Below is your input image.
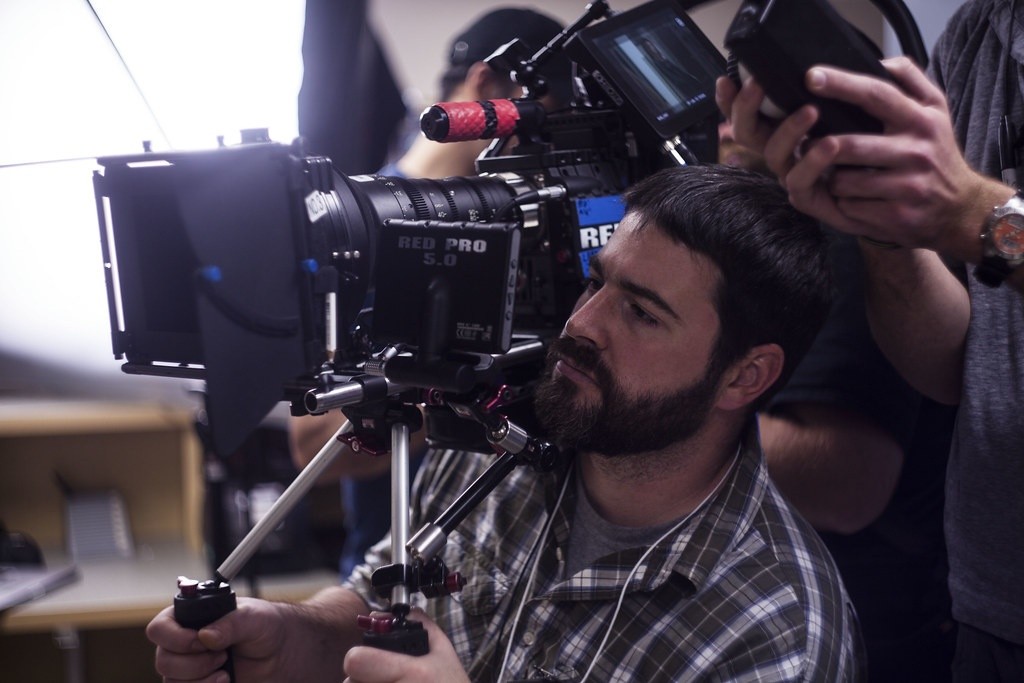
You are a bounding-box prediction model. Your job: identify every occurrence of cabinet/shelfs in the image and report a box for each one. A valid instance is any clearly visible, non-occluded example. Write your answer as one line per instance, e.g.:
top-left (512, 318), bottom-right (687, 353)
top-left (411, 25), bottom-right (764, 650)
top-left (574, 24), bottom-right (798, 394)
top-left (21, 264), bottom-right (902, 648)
top-left (0, 401), bottom-right (343, 683)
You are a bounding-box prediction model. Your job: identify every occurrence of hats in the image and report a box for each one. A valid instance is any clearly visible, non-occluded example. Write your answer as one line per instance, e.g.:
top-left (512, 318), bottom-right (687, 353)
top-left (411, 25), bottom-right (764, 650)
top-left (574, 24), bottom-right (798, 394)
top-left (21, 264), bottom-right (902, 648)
top-left (448, 8), bottom-right (567, 71)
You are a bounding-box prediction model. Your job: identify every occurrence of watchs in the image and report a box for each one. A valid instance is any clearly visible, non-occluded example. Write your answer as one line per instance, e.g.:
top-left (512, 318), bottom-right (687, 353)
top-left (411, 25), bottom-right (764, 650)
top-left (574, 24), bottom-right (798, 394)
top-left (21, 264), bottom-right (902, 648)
top-left (972, 188), bottom-right (1024, 289)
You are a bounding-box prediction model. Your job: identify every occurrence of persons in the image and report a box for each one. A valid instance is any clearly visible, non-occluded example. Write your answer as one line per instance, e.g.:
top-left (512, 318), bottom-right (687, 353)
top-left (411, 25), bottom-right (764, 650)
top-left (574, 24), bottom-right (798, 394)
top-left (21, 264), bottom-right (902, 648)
top-left (146, 164), bottom-right (861, 683)
top-left (715, 0), bottom-right (1024, 683)
top-left (287, 6), bottom-right (565, 585)
